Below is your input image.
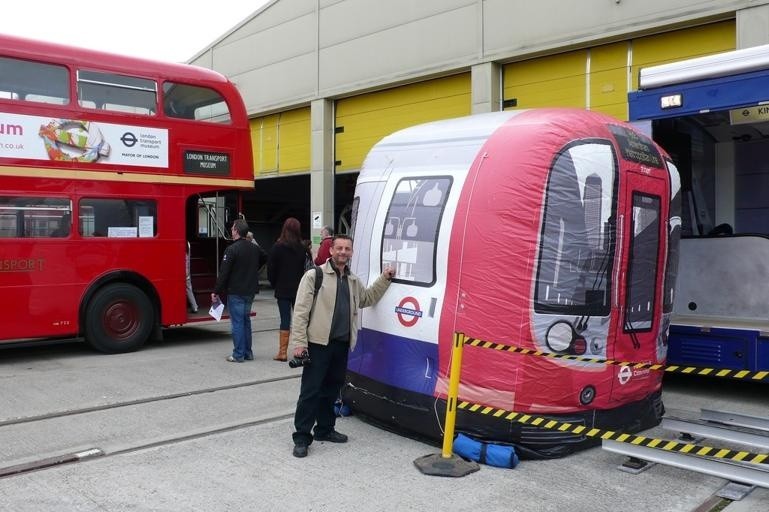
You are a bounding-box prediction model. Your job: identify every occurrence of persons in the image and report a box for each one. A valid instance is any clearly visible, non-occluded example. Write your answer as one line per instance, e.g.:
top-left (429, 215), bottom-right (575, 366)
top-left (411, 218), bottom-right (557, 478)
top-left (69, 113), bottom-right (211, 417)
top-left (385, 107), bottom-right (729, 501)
top-left (209, 218), bottom-right (265, 362)
top-left (245, 231), bottom-right (259, 249)
top-left (186, 240), bottom-right (199, 316)
top-left (49, 213), bottom-right (71, 238)
top-left (291, 236), bottom-right (395, 460)
top-left (314, 224), bottom-right (335, 264)
top-left (266, 213), bottom-right (310, 360)
top-left (304, 238), bottom-right (314, 269)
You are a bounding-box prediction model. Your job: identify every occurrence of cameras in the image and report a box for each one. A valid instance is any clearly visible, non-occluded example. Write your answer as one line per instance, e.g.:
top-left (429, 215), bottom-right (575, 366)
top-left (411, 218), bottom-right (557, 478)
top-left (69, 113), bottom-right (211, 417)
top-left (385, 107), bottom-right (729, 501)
top-left (289, 352), bottom-right (311, 368)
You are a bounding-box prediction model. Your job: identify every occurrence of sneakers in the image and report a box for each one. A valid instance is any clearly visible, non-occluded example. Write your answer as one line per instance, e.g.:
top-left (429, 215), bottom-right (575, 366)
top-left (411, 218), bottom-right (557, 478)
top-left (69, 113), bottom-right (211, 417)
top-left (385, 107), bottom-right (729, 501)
top-left (226, 355), bottom-right (238, 362)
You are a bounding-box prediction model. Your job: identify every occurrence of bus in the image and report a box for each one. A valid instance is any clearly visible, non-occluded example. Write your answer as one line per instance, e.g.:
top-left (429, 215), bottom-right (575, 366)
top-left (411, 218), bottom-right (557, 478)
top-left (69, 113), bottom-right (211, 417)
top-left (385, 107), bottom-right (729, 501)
top-left (0, 30), bottom-right (260, 357)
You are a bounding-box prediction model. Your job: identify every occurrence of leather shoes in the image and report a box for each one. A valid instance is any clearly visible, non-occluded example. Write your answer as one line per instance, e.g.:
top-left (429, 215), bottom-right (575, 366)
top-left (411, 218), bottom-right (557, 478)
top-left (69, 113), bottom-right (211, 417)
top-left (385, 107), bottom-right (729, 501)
top-left (313, 428), bottom-right (348, 443)
top-left (293, 443), bottom-right (308, 457)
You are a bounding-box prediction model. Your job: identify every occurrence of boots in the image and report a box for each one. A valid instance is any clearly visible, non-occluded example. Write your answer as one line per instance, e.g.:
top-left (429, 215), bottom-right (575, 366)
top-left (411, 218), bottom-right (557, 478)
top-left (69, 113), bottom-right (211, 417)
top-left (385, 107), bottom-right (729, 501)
top-left (273, 329), bottom-right (290, 362)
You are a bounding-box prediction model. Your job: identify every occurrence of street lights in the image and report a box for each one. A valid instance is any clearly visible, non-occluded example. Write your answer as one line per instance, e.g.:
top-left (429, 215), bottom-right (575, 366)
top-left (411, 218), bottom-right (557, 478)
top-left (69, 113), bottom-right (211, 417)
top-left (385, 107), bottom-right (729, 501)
top-left (383, 215), bottom-right (421, 277)
